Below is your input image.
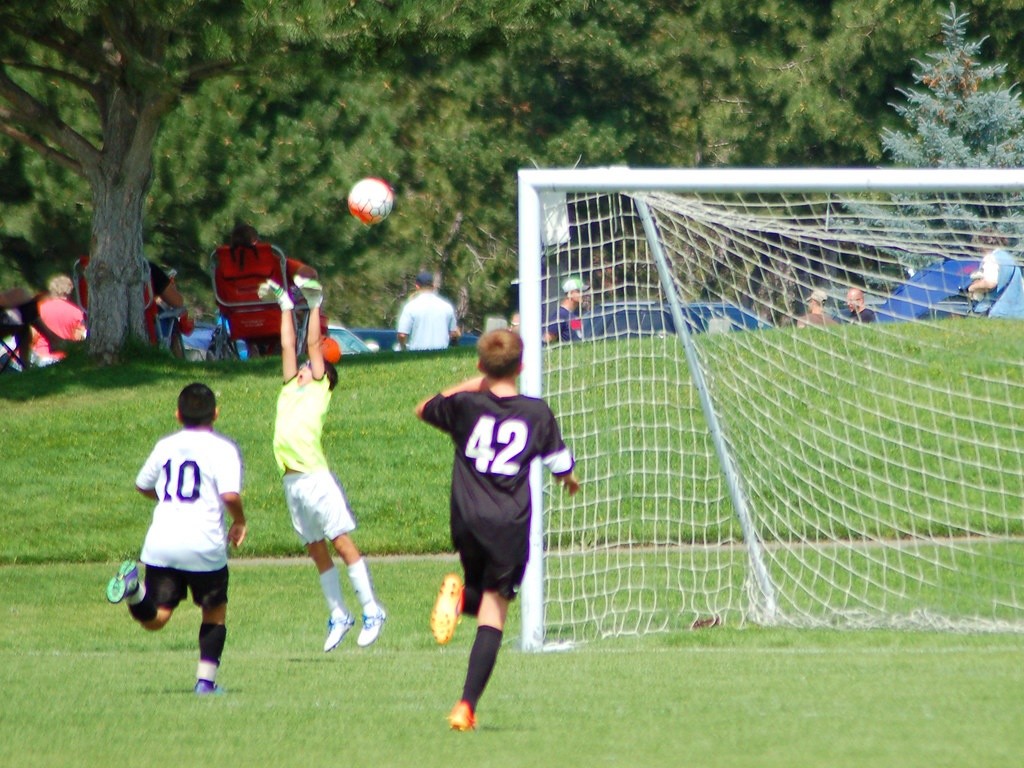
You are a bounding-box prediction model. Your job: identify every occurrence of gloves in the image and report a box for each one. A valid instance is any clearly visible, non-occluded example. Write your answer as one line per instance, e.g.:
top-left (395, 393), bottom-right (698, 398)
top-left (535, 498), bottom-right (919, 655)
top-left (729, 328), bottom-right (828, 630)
top-left (258, 279), bottom-right (294, 312)
top-left (293, 274), bottom-right (324, 307)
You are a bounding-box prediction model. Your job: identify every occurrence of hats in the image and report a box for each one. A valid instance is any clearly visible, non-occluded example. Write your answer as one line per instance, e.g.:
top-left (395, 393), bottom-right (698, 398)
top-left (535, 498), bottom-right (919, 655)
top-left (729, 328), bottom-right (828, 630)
top-left (806, 291), bottom-right (827, 303)
top-left (415, 271), bottom-right (433, 286)
top-left (562, 278), bottom-right (591, 293)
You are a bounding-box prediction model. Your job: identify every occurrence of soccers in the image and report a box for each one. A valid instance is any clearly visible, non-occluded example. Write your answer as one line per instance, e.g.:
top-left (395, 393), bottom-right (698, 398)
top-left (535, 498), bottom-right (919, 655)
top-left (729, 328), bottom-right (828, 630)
top-left (347, 177), bottom-right (394, 225)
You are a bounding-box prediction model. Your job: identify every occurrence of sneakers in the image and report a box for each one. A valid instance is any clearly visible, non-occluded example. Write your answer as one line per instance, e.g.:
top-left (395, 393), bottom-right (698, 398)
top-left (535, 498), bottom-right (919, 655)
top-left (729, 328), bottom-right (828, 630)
top-left (194, 679), bottom-right (228, 699)
top-left (323, 610), bottom-right (355, 653)
top-left (357, 606), bottom-right (386, 647)
top-left (429, 573), bottom-right (465, 644)
top-left (444, 701), bottom-right (478, 735)
top-left (105, 559), bottom-right (139, 604)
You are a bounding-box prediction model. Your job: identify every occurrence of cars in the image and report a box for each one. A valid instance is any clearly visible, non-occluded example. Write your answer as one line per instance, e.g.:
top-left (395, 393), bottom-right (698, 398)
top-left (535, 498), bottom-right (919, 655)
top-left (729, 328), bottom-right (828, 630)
top-left (557, 254), bottom-right (979, 341)
top-left (183, 322), bottom-right (482, 361)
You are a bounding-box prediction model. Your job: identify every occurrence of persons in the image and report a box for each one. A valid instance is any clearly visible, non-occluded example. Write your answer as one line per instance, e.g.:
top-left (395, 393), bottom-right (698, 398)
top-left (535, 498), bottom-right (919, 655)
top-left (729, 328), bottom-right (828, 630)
top-left (148, 262), bottom-right (184, 340)
top-left (107, 383), bottom-right (248, 694)
top-left (397, 273), bottom-right (462, 349)
top-left (257, 274), bottom-right (387, 653)
top-left (967, 224), bottom-right (1024, 319)
top-left (846, 288), bottom-right (874, 323)
top-left (14, 276), bottom-right (86, 372)
top-left (414, 329), bottom-right (579, 732)
top-left (543, 278), bottom-right (591, 343)
top-left (797, 291), bottom-right (837, 328)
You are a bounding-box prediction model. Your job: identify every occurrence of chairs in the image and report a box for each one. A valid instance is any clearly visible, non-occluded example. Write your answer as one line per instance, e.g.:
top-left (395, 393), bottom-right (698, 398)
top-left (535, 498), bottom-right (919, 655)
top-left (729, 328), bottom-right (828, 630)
top-left (209, 243), bottom-right (329, 359)
top-left (1, 257), bottom-right (187, 374)
